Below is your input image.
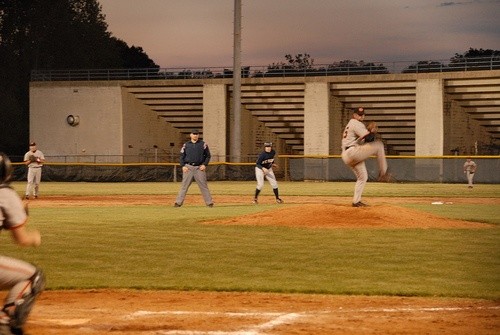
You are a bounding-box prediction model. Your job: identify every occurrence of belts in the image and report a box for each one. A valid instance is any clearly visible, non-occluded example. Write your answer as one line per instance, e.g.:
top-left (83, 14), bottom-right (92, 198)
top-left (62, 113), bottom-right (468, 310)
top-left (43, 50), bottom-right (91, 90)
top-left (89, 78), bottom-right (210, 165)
top-left (33, 167), bottom-right (40, 168)
top-left (184, 162), bottom-right (199, 166)
top-left (346, 147), bottom-right (349, 149)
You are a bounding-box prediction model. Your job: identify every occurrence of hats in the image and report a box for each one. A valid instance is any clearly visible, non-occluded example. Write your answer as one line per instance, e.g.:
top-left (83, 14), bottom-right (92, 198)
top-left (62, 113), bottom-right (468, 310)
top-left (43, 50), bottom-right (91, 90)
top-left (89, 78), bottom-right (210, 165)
top-left (29, 142), bottom-right (35, 145)
top-left (354, 107), bottom-right (366, 114)
top-left (192, 129), bottom-right (199, 133)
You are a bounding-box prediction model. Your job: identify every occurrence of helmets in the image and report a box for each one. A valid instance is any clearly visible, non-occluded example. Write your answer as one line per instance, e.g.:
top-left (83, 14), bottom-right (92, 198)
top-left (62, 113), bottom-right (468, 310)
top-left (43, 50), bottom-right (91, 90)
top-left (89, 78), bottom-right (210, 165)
top-left (264, 141), bottom-right (273, 147)
top-left (0, 152), bottom-right (13, 184)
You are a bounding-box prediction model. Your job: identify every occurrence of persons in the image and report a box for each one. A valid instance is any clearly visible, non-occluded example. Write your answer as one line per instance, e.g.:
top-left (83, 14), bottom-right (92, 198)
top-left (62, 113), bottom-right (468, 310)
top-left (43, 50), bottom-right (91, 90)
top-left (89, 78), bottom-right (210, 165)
top-left (463, 157), bottom-right (476, 188)
top-left (252, 142), bottom-right (282, 205)
top-left (0, 152), bottom-right (46, 335)
top-left (174, 130), bottom-right (214, 208)
top-left (341, 107), bottom-right (394, 207)
top-left (24, 142), bottom-right (45, 200)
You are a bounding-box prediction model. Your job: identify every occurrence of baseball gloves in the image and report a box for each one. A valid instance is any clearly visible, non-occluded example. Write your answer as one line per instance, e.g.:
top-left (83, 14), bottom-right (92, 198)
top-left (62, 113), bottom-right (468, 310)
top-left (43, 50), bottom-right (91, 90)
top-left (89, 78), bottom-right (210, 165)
top-left (29, 154), bottom-right (36, 161)
top-left (272, 163), bottom-right (279, 172)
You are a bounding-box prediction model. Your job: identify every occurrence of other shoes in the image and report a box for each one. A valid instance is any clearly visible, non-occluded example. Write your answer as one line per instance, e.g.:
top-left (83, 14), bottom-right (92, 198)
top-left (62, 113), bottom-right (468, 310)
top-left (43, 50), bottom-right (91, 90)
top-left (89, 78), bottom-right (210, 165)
top-left (0, 326), bottom-right (23, 335)
top-left (253, 200), bottom-right (258, 203)
top-left (376, 175), bottom-right (392, 180)
top-left (276, 198), bottom-right (282, 203)
top-left (34, 195), bottom-right (37, 198)
top-left (208, 203), bottom-right (213, 207)
top-left (174, 203), bottom-right (181, 207)
top-left (26, 195), bottom-right (29, 199)
top-left (352, 201), bottom-right (366, 207)
top-left (468, 186), bottom-right (473, 188)
